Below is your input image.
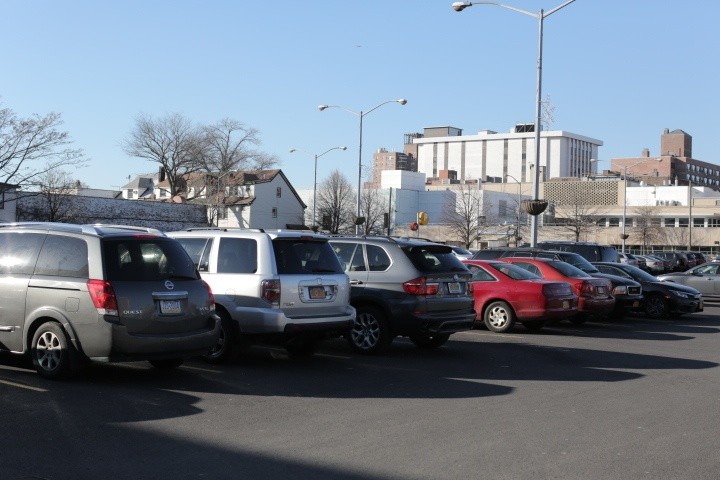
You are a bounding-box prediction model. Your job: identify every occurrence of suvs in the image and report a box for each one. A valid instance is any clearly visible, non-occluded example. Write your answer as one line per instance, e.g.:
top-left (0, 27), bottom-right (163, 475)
top-left (304, 233), bottom-right (478, 357)
top-left (470, 245), bottom-right (646, 325)
top-left (120, 226), bottom-right (357, 357)
top-left (0, 221), bottom-right (221, 383)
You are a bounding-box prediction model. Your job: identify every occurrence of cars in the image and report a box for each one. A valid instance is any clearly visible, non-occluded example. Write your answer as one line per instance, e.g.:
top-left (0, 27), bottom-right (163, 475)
top-left (572, 261), bottom-right (705, 321)
top-left (462, 259), bottom-right (580, 333)
top-left (423, 240), bottom-right (720, 277)
top-left (654, 259), bottom-right (720, 302)
top-left (473, 256), bottom-right (616, 326)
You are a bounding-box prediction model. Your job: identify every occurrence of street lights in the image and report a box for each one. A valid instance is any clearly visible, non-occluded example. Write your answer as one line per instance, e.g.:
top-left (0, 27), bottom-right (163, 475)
top-left (216, 170), bottom-right (238, 228)
top-left (504, 173), bottom-right (521, 247)
top-left (288, 145), bottom-right (347, 226)
top-left (452, 0), bottom-right (578, 247)
top-left (591, 157), bottom-right (663, 253)
top-left (318, 98), bottom-right (408, 234)
